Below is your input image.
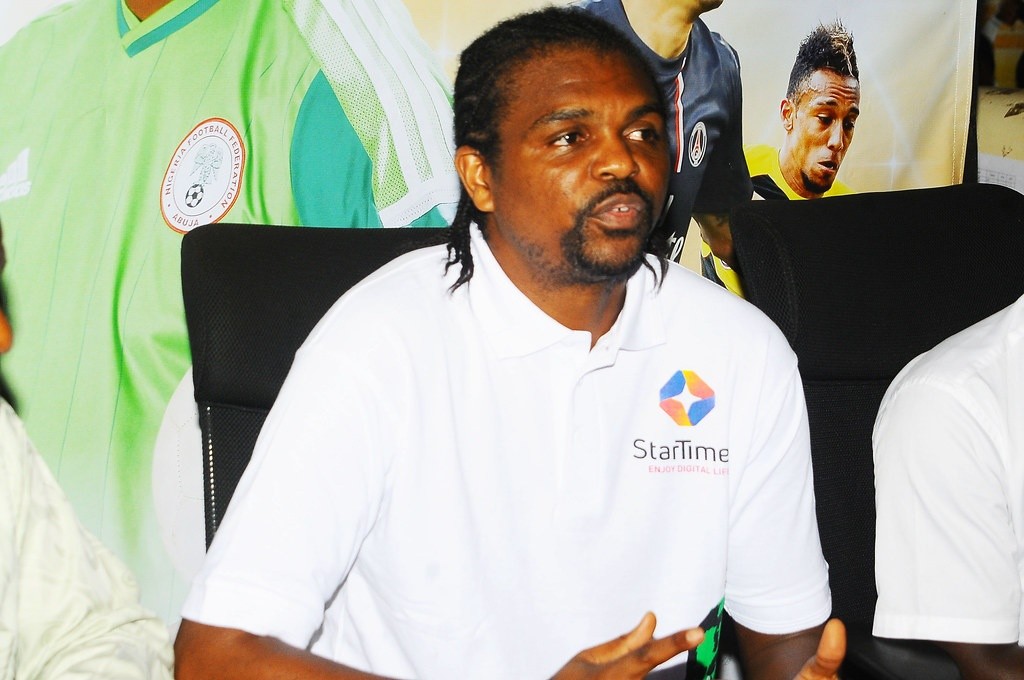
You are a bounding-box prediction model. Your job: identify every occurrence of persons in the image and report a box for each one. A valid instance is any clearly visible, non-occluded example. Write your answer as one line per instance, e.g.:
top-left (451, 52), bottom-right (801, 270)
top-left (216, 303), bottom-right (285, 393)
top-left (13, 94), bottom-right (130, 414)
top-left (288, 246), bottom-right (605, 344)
top-left (0, 306), bottom-right (174, 680)
top-left (872, 294), bottom-right (1024, 680)
top-left (0, 0), bottom-right (861, 631)
top-left (173, 7), bottom-right (846, 680)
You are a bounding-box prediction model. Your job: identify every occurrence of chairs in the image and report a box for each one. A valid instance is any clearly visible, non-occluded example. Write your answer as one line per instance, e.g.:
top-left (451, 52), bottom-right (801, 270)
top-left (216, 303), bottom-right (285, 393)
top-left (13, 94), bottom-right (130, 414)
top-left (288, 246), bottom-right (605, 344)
top-left (723, 183), bottom-right (1024, 680)
top-left (180, 222), bottom-right (465, 556)
